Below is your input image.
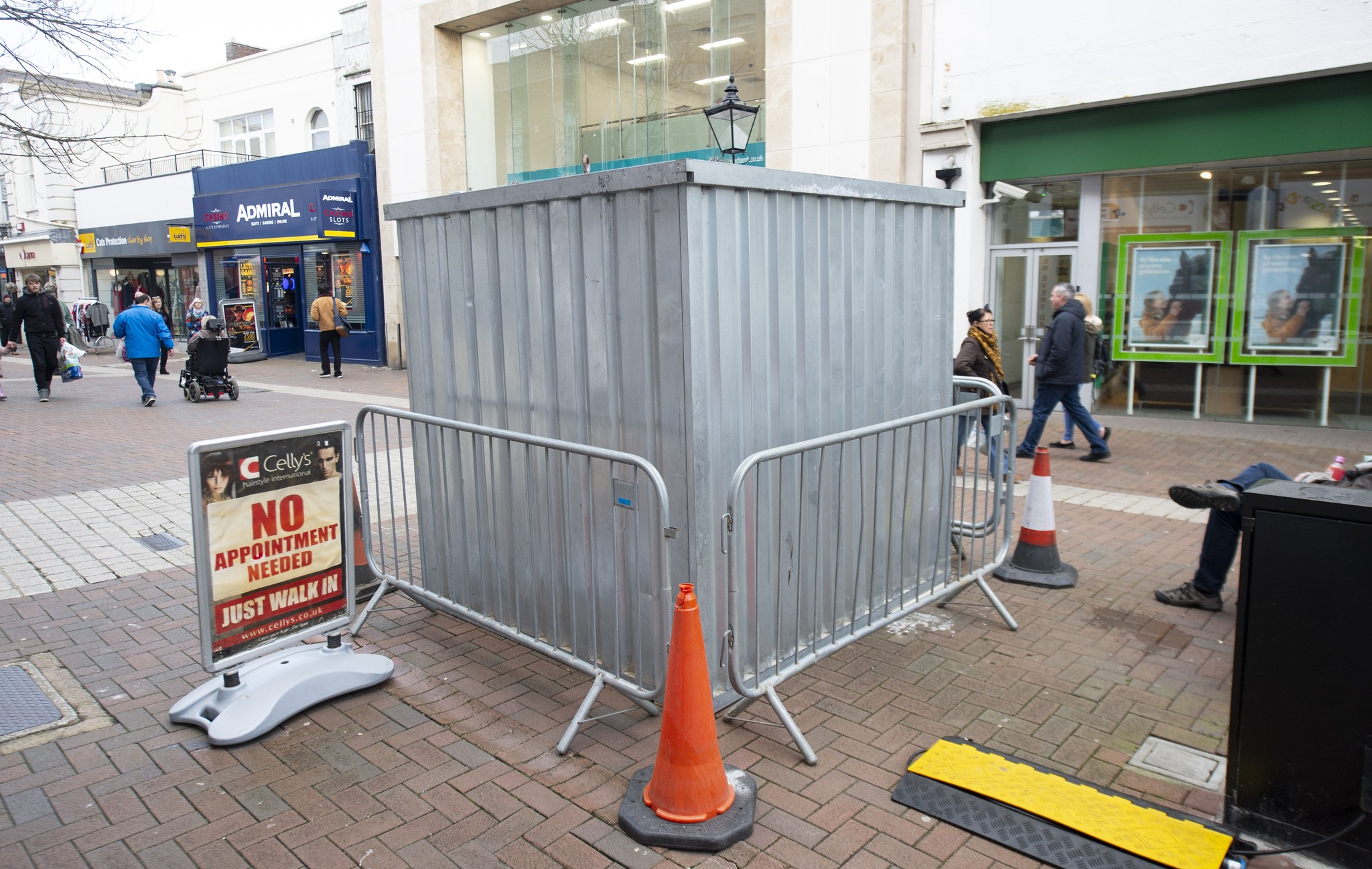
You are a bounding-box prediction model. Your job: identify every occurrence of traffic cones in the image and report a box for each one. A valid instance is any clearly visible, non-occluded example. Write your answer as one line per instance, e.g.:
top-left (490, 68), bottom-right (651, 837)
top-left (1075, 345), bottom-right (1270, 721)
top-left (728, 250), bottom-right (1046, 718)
top-left (618, 584), bottom-right (757, 852)
top-left (993, 446), bottom-right (1078, 589)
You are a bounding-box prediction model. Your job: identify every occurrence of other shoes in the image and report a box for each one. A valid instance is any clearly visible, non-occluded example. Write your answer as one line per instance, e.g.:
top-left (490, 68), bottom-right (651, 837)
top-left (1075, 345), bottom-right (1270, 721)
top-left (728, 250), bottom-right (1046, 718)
top-left (160, 367), bottom-right (170, 375)
top-left (1004, 447), bottom-right (1034, 459)
top-left (953, 468), bottom-right (963, 476)
top-left (1090, 427), bottom-right (1111, 449)
top-left (992, 475), bottom-right (1021, 484)
top-left (1080, 451), bottom-right (1111, 461)
top-left (1050, 441), bottom-right (1075, 450)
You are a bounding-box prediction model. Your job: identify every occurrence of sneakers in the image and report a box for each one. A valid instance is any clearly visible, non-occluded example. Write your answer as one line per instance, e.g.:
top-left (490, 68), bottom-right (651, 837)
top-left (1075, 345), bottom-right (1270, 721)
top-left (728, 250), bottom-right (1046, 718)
top-left (39, 389), bottom-right (49, 402)
top-left (318, 371), bottom-right (331, 377)
top-left (332, 373), bottom-right (342, 378)
top-left (143, 397), bottom-right (156, 406)
top-left (1155, 580), bottom-right (1223, 611)
top-left (1169, 483), bottom-right (1240, 512)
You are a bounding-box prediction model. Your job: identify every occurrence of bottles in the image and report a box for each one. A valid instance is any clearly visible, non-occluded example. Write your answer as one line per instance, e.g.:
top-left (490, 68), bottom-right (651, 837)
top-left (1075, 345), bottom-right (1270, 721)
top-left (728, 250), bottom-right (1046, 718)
top-left (1322, 455), bottom-right (1346, 482)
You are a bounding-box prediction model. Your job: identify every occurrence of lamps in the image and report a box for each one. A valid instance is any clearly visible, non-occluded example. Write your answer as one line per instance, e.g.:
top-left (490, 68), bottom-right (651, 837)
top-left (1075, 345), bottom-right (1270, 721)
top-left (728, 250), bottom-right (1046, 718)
top-left (110, 270), bottom-right (119, 276)
top-left (48, 269), bottom-right (59, 283)
top-left (16, 222), bottom-right (25, 232)
top-left (156, 270), bottom-right (164, 276)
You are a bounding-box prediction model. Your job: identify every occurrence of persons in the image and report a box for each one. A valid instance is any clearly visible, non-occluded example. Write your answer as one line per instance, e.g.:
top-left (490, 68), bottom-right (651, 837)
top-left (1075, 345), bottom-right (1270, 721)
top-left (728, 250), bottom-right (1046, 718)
top-left (311, 283), bottom-right (348, 378)
top-left (1262, 290), bottom-right (1310, 355)
top-left (1005, 282), bottom-right (1112, 462)
top-left (126, 292), bottom-right (174, 374)
top-left (311, 438), bottom-right (343, 483)
top-left (44, 288), bottom-right (75, 375)
top-left (200, 452), bottom-right (236, 515)
top-left (952, 308), bottom-right (1022, 483)
top-left (113, 294), bottom-right (174, 407)
top-left (1140, 290), bottom-right (1182, 351)
top-left (0, 294), bottom-right (19, 355)
top-left (187, 315), bottom-right (231, 380)
top-left (231, 305), bottom-right (246, 323)
top-left (121, 281), bottom-right (133, 309)
top-left (186, 298), bottom-right (210, 347)
top-left (0, 343), bottom-right (13, 400)
top-left (7, 273), bottom-right (67, 402)
top-left (1155, 454), bottom-right (1372, 611)
top-left (337, 256), bottom-right (351, 285)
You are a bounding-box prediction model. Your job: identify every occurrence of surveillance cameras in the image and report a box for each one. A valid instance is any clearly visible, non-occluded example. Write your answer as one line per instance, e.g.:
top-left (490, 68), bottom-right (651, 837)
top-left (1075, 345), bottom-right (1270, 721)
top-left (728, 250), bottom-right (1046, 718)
top-left (991, 179), bottom-right (1031, 200)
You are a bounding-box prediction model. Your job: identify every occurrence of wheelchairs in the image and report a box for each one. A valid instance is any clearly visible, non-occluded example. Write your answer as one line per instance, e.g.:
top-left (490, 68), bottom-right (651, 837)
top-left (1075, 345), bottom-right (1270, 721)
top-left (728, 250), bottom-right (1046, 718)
top-left (179, 318), bottom-right (239, 403)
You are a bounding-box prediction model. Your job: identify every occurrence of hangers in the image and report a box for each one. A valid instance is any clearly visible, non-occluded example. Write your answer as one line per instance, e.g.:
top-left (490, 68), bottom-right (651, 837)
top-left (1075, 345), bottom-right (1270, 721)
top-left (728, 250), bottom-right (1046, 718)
top-left (75, 296), bottom-right (101, 307)
top-left (161, 278), bottom-right (174, 287)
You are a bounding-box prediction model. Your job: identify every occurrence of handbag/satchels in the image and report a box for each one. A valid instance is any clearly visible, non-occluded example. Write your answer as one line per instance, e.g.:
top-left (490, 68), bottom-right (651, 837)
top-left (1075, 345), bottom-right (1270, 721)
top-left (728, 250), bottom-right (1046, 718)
top-left (334, 315), bottom-right (353, 338)
top-left (115, 337), bottom-right (129, 364)
top-left (58, 341), bottom-right (86, 383)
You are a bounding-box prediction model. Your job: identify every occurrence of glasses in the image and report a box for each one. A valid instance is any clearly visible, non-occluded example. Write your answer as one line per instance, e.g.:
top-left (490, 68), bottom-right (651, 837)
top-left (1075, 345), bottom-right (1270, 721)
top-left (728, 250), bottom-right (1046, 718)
top-left (978, 320), bottom-right (995, 324)
top-left (152, 296), bottom-right (160, 298)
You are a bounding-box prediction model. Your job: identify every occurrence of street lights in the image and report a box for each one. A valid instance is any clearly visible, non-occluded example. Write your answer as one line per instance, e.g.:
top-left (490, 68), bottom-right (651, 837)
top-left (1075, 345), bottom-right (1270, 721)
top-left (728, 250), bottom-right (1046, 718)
top-left (703, 71), bottom-right (760, 165)
